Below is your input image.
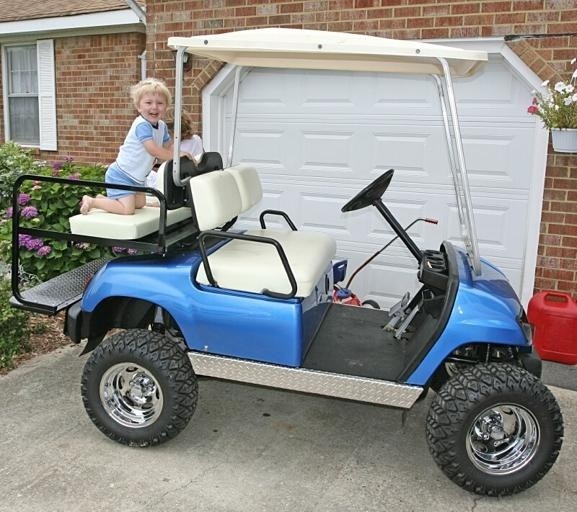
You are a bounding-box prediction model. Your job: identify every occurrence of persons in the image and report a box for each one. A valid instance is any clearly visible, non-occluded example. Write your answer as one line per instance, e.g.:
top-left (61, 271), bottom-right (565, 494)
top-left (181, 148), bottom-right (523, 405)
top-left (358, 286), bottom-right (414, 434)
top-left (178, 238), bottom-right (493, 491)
top-left (80, 78), bottom-right (197, 218)
top-left (146, 106), bottom-right (203, 207)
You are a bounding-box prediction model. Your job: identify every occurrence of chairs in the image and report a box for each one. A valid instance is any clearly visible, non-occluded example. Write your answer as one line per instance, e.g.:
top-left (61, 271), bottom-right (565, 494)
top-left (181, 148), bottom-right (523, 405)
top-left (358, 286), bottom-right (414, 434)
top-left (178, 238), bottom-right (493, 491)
top-left (69, 147), bottom-right (224, 239)
top-left (188, 162), bottom-right (337, 300)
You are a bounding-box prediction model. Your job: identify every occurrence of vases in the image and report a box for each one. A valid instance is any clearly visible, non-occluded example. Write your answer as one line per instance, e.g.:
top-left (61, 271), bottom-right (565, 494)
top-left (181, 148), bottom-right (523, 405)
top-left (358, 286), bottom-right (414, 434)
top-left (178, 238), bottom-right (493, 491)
top-left (551, 128), bottom-right (577, 153)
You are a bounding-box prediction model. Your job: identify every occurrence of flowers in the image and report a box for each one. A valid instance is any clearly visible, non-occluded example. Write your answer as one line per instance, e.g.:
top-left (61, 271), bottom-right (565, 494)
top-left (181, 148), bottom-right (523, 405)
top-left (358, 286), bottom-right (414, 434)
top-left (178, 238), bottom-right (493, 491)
top-left (526, 57), bottom-right (577, 130)
